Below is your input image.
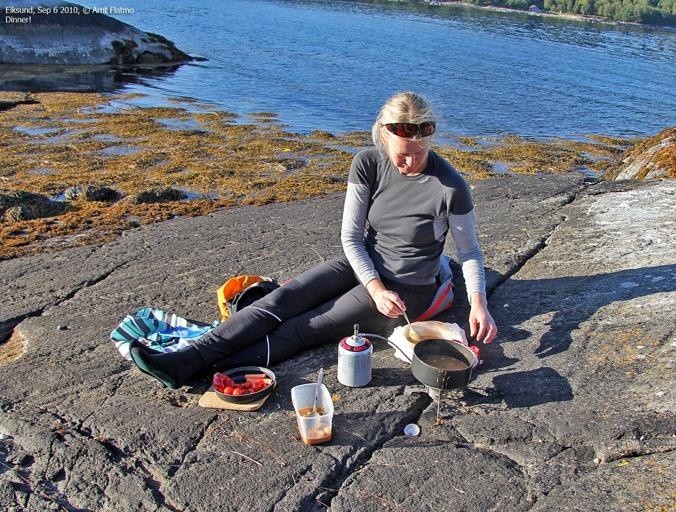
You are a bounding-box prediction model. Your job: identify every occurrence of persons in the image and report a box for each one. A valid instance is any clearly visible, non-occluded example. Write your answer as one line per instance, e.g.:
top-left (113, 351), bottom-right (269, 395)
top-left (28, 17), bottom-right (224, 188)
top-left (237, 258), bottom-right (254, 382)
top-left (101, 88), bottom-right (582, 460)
top-left (128, 90), bottom-right (500, 393)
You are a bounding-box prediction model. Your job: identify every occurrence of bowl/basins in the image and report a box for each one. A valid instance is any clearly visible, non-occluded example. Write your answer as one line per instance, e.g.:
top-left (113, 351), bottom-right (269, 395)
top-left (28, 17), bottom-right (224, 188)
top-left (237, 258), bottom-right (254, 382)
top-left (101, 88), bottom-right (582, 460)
top-left (410, 339), bottom-right (477, 389)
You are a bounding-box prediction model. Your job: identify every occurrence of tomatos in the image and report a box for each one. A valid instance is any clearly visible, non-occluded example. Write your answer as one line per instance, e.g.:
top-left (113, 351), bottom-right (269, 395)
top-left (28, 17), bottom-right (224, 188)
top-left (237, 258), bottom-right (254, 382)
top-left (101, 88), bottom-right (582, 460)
top-left (223, 380), bottom-right (266, 396)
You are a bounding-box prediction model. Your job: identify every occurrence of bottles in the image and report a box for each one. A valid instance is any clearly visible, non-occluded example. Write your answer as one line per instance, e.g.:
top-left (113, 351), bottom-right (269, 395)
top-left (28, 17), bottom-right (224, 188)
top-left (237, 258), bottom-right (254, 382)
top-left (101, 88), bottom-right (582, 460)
top-left (337, 324), bottom-right (372, 388)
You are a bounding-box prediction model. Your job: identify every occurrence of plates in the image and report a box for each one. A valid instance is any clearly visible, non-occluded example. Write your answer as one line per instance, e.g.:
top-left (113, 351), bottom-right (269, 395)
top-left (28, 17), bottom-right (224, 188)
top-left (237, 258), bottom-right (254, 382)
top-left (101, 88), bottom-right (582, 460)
top-left (212, 367), bottom-right (276, 403)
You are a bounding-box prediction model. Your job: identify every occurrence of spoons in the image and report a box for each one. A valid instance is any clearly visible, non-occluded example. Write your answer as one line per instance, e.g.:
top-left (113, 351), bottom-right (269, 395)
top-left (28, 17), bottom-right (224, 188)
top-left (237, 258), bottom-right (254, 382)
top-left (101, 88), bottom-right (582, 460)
top-left (305, 367), bottom-right (326, 424)
top-left (398, 300), bottom-right (423, 342)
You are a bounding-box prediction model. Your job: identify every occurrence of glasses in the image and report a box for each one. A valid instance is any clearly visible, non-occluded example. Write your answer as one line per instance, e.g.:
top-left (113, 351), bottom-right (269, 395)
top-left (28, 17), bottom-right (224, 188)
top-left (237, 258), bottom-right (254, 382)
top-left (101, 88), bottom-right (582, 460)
top-left (380, 120), bottom-right (437, 138)
